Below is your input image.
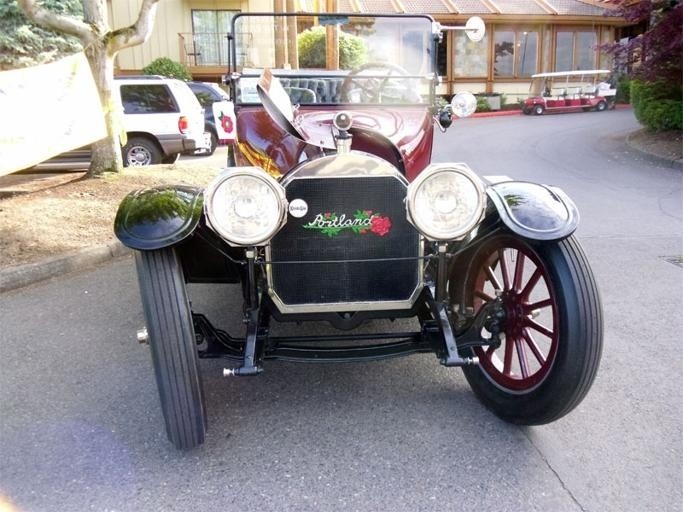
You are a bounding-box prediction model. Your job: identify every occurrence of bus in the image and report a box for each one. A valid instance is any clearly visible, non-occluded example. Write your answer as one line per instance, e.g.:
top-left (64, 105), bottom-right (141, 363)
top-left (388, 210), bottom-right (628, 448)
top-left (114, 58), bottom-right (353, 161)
top-left (521, 69), bottom-right (616, 114)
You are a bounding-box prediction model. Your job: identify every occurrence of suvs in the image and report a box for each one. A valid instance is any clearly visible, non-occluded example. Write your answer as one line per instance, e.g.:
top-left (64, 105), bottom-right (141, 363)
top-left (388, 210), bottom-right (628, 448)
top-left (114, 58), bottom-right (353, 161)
top-left (184, 80), bottom-right (232, 156)
top-left (114, 11), bottom-right (606, 455)
top-left (16, 76), bottom-right (211, 169)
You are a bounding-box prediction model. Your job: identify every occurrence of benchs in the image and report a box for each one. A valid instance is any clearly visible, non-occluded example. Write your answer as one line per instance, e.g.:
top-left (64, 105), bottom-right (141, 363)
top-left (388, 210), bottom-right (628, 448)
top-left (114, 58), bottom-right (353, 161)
top-left (548, 86), bottom-right (599, 100)
top-left (279, 78), bottom-right (421, 105)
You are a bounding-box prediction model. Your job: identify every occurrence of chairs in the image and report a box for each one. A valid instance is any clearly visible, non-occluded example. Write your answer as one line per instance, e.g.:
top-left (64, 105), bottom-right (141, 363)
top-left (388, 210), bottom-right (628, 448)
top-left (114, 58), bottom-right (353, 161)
top-left (283, 87), bottom-right (316, 104)
top-left (347, 87), bottom-right (420, 105)
